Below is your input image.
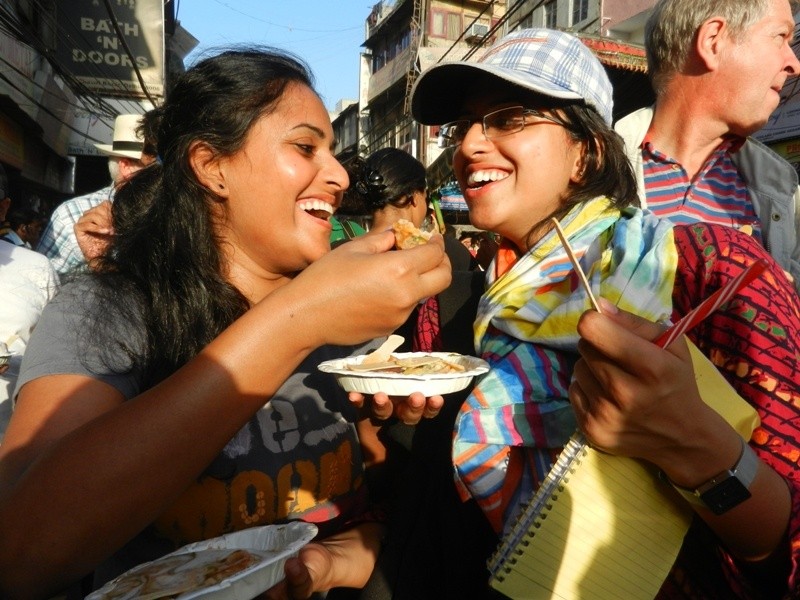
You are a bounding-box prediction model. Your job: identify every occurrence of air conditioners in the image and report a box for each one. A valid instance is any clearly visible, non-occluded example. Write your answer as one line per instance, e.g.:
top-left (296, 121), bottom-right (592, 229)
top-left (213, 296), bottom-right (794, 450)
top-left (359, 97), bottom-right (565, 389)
top-left (463, 23), bottom-right (489, 40)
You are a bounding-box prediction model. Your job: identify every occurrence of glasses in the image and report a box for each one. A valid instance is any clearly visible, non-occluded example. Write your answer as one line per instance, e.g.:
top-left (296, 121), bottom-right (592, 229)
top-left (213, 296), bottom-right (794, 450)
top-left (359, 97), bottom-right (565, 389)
top-left (435, 105), bottom-right (584, 149)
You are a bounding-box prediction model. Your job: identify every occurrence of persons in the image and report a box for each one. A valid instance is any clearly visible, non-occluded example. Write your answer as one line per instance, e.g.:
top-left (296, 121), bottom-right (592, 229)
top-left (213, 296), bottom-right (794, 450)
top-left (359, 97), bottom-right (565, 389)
top-left (0, 41), bottom-right (451, 600)
top-left (249, 26), bottom-right (800, 600)
top-left (0, 0), bottom-right (800, 600)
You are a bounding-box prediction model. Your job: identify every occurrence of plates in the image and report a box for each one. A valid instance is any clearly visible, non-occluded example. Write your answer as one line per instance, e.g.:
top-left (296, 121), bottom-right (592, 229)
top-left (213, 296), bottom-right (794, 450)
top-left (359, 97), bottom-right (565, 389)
top-left (86, 521), bottom-right (319, 599)
top-left (317, 352), bottom-right (492, 397)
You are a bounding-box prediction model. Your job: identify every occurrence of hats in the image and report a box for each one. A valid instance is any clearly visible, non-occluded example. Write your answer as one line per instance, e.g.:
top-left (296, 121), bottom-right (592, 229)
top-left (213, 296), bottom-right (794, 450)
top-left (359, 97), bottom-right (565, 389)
top-left (93, 114), bottom-right (148, 159)
top-left (408, 28), bottom-right (614, 130)
